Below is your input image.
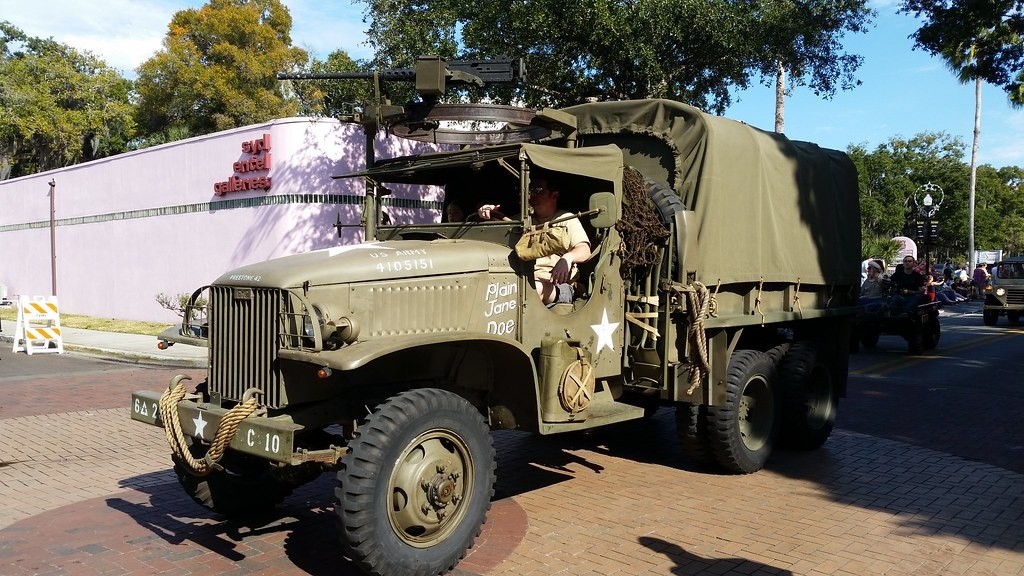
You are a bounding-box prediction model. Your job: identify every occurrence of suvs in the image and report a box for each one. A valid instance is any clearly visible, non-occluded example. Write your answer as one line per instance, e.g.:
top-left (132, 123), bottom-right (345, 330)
top-left (983, 256), bottom-right (1024, 325)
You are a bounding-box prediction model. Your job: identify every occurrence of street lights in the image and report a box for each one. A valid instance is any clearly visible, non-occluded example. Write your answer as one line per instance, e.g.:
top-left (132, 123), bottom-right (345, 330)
top-left (914, 181), bottom-right (945, 279)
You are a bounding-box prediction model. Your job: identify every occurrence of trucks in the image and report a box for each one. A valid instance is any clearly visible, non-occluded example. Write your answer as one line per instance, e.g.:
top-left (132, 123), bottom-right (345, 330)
top-left (131, 52), bottom-right (865, 576)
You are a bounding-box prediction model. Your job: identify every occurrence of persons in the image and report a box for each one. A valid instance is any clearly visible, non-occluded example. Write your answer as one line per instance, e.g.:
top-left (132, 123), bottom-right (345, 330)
top-left (861, 256), bottom-right (1024, 314)
top-left (445, 170), bottom-right (592, 305)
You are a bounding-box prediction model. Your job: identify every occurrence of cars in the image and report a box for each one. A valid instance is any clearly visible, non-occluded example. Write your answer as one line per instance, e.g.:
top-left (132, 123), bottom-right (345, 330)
top-left (933, 265), bottom-right (949, 277)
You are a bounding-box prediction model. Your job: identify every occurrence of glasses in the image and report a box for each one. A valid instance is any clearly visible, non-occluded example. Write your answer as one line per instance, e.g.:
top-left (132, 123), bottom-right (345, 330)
top-left (530, 186), bottom-right (549, 194)
top-left (867, 266), bottom-right (874, 270)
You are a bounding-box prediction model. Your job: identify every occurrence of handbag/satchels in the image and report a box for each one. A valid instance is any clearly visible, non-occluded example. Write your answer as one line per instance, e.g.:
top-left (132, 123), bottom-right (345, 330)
top-left (515, 221), bottom-right (570, 261)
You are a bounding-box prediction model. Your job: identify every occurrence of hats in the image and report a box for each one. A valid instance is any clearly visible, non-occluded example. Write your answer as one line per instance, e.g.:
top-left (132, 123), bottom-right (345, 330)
top-left (868, 260), bottom-right (884, 274)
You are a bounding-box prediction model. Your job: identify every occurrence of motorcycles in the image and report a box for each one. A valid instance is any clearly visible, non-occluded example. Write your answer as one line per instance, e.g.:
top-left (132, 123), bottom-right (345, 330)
top-left (865, 284), bottom-right (941, 354)
